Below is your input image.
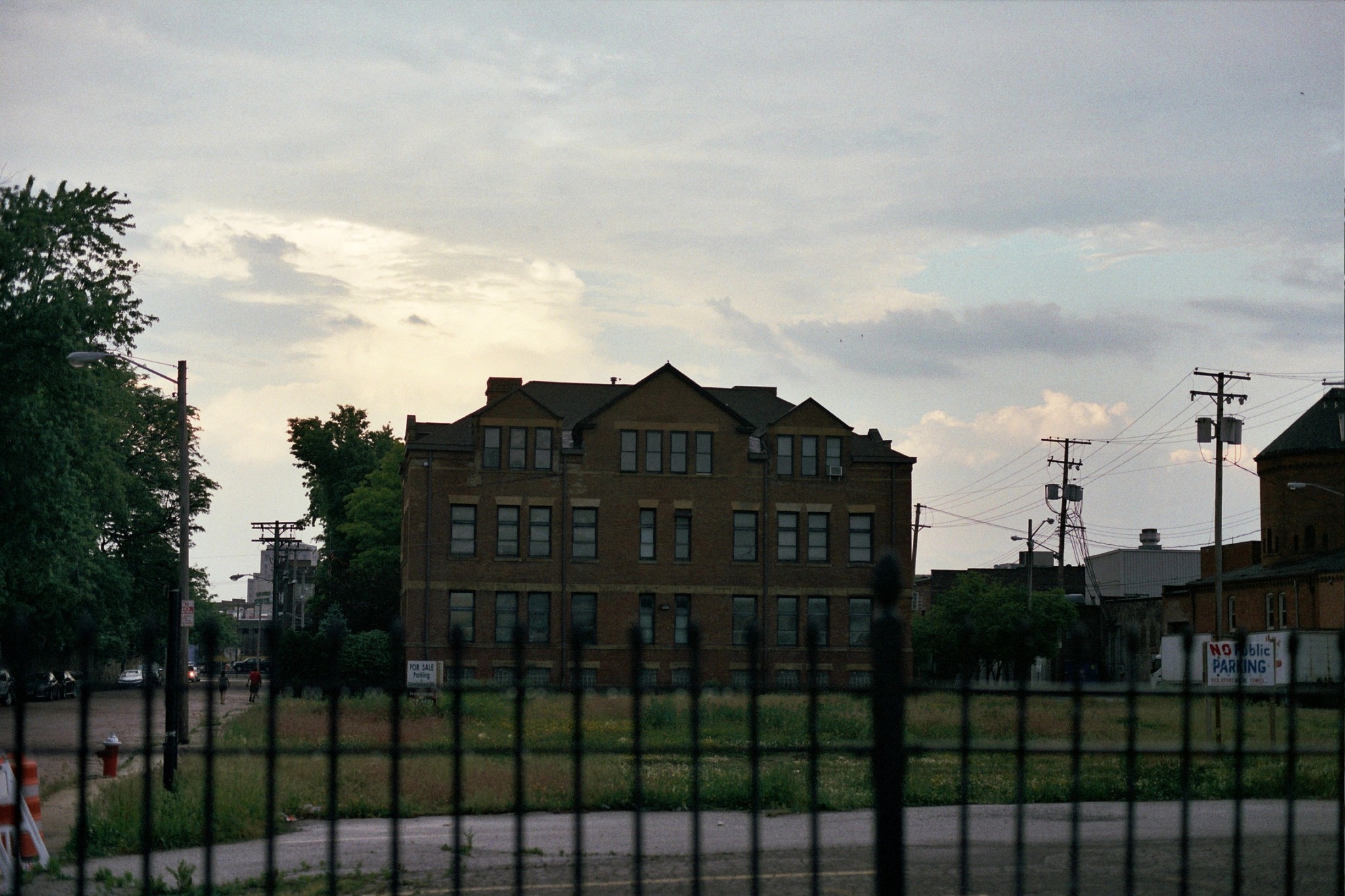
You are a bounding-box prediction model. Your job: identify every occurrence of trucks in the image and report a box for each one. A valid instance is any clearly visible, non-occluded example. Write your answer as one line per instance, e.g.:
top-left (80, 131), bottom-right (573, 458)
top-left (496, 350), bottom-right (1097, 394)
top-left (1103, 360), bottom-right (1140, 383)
top-left (1150, 633), bottom-right (1234, 686)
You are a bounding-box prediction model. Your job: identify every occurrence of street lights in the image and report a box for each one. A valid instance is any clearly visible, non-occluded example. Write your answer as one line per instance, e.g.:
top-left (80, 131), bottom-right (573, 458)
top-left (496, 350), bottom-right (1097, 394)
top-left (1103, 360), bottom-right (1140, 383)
top-left (1028, 517), bottom-right (1055, 613)
top-left (65, 350), bottom-right (187, 791)
top-left (279, 612), bottom-right (304, 629)
top-left (230, 573), bottom-right (278, 629)
top-left (1011, 535), bottom-right (1064, 595)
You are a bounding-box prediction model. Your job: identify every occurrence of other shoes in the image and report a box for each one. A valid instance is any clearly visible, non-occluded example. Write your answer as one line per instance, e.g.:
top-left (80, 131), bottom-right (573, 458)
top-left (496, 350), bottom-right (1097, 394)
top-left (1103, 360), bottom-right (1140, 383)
top-left (249, 697), bottom-right (252, 702)
top-left (252, 699), bottom-right (254, 702)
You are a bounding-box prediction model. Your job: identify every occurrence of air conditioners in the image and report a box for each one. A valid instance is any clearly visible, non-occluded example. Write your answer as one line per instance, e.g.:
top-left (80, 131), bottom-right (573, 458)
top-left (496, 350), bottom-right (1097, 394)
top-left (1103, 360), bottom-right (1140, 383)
top-left (826, 465), bottom-right (844, 477)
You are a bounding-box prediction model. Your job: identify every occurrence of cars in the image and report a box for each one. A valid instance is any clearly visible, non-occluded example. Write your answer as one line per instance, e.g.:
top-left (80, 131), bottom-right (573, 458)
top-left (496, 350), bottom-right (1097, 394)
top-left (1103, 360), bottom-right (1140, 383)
top-left (155, 668), bottom-right (162, 687)
top-left (117, 669), bottom-right (143, 687)
top-left (0, 670), bottom-right (77, 707)
top-left (188, 664), bottom-right (201, 682)
top-left (232, 657), bottom-right (270, 673)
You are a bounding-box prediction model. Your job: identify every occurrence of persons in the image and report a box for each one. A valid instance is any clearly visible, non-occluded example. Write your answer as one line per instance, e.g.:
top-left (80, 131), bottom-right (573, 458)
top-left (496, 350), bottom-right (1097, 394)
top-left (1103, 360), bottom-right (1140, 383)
top-left (246, 667), bottom-right (262, 703)
top-left (216, 670), bottom-right (230, 704)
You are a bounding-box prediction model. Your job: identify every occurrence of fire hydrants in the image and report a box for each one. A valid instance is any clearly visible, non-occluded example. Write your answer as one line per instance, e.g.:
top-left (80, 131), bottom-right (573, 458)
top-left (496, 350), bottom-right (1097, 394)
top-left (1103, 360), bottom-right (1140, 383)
top-left (95, 731), bottom-right (123, 778)
